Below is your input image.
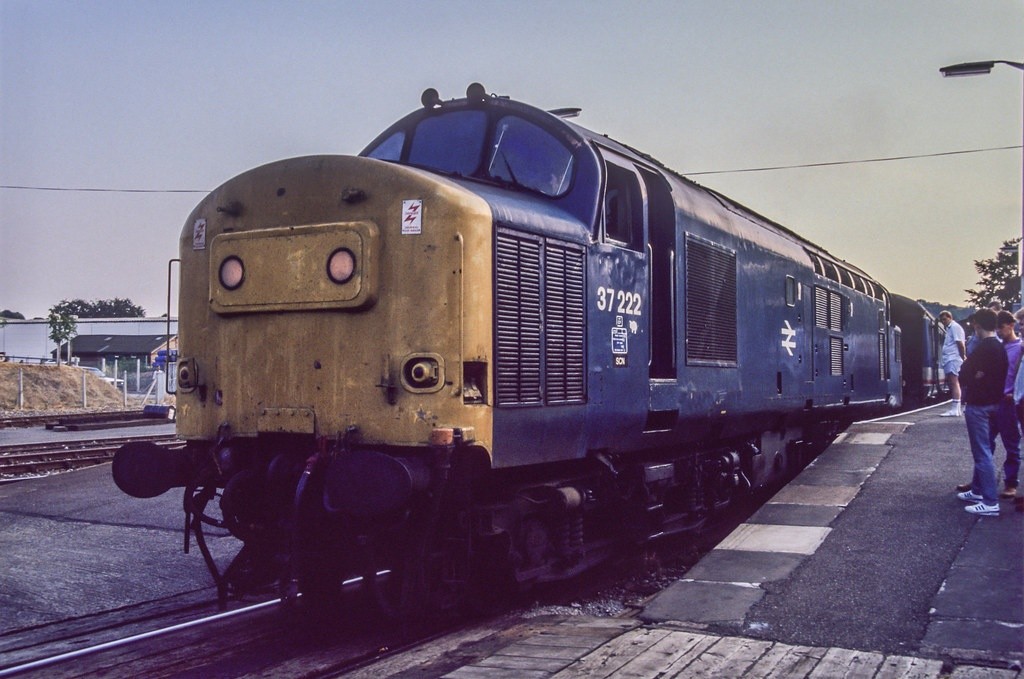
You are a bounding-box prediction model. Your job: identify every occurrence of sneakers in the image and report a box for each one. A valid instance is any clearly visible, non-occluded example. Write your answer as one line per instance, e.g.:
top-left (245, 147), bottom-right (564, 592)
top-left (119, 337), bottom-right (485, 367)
top-left (964, 501), bottom-right (1000, 516)
top-left (957, 490), bottom-right (983, 504)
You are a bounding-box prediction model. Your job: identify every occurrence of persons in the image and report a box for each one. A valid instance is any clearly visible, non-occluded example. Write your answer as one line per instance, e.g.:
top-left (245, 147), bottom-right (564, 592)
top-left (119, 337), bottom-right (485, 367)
top-left (957, 300), bottom-right (1024, 516)
top-left (152, 365), bottom-right (164, 380)
top-left (938, 310), bottom-right (967, 417)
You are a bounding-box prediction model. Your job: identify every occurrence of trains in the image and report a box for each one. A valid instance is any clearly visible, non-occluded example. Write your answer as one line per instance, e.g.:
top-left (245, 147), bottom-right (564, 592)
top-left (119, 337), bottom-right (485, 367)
top-left (110, 82), bottom-right (946, 616)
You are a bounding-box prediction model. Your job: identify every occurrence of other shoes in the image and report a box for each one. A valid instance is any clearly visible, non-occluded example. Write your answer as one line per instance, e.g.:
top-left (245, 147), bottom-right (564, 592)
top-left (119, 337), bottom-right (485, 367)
top-left (940, 408), bottom-right (961, 416)
top-left (957, 484), bottom-right (971, 492)
top-left (1001, 486), bottom-right (1016, 497)
